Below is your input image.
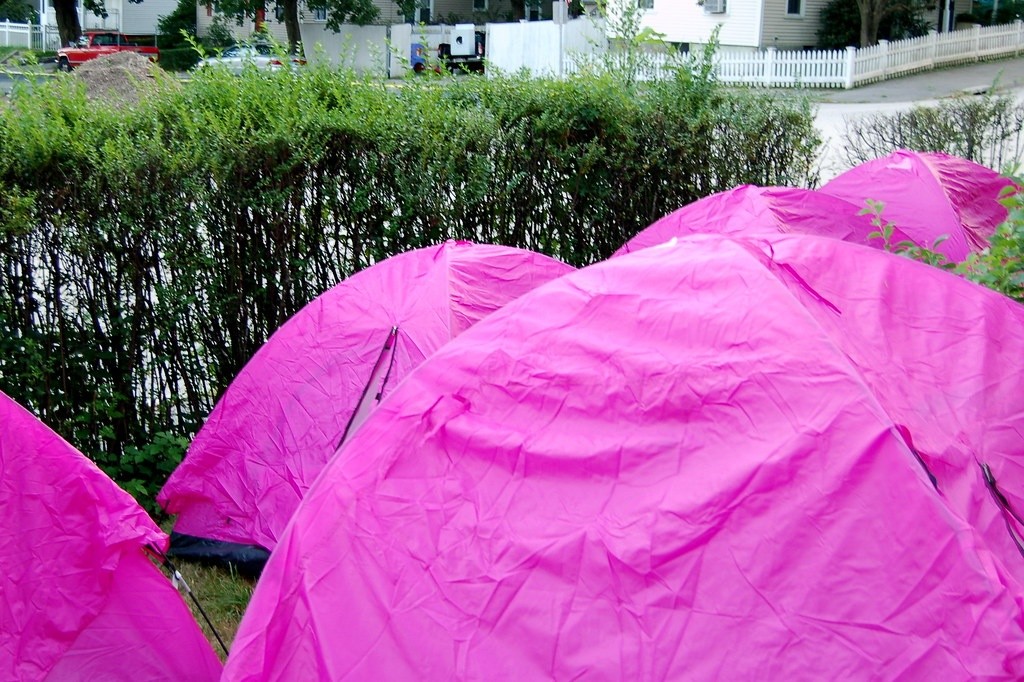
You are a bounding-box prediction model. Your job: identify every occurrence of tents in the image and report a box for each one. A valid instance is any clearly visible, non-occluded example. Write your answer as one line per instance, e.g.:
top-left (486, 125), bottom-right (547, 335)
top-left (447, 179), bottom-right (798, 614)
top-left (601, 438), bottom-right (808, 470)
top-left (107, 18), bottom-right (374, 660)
top-left (156, 148), bottom-right (1024, 682)
top-left (0, 388), bottom-right (230, 682)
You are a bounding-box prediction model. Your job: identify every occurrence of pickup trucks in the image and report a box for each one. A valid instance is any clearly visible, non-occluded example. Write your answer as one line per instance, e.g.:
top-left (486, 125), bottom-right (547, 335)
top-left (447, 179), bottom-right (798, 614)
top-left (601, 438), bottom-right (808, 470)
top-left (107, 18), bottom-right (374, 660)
top-left (438, 29), bottom-right (485, 75)
top-left (54, 30), bottom-right (159, 75)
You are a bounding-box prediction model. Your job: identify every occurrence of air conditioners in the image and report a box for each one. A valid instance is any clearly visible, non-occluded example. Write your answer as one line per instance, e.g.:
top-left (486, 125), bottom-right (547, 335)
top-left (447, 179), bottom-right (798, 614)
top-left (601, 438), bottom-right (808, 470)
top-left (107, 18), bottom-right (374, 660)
top-left (704, 0), bottom-right (724, 13)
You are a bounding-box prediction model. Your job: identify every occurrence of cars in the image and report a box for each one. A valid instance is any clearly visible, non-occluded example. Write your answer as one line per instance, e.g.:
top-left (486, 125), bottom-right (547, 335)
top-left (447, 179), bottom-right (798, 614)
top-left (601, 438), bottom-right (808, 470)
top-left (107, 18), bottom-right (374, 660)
top-left (195, 42), bottom-right (307, 81)
